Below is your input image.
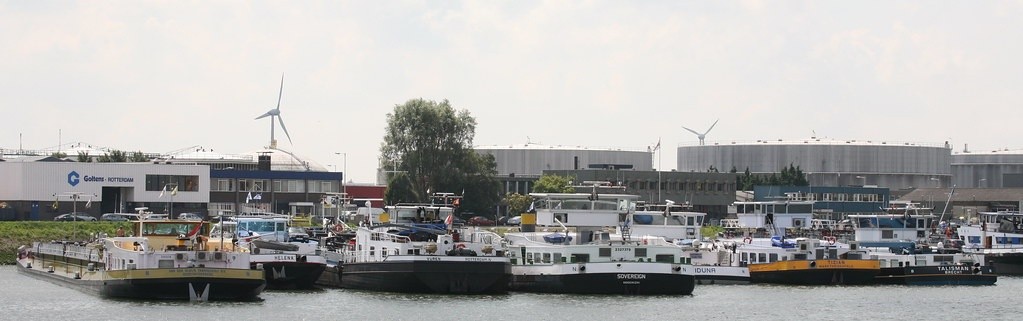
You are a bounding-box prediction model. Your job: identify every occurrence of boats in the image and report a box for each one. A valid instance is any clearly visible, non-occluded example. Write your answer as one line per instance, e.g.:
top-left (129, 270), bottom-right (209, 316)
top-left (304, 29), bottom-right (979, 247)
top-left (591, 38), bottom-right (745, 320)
top-left (130, 180), bottom-right (1023, 297)
top-left (13, 207), bottom-right (266, 303)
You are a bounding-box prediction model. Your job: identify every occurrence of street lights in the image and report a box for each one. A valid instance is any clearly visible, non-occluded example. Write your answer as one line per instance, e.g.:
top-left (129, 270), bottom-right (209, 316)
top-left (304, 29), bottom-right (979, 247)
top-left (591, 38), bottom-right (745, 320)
top-left (979, 178), bottom-right (987, 187)
top-left (931, 177), bottom-right (940, 187)
top-left (856, 175), bottom-right (866, 185)
top-left (335, 153), bottom-right (346, 203)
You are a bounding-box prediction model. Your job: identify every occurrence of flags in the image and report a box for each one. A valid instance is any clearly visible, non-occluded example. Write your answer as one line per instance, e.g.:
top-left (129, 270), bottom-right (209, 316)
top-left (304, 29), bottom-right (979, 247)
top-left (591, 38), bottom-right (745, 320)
top-left (85, 197), bottom-right (92, 209)
top-left (52, 197), bottom-right (58, 210)
top-left (171, 185), bottom-right (178, 196)
top-left (444, 215), bottom-right (453, 226)
top-left (246, 192), bottom-right (262, 204)
top-left (158, 185), bottom-right (166, 199)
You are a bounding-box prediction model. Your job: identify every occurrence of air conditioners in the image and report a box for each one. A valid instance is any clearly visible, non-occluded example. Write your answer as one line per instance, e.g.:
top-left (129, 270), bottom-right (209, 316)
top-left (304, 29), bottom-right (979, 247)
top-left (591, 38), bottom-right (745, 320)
top-left (212, 251), bottom-right (227, 261)
top-left (197, 251), bottom-right (210, 261)
top-left (174, 253), bottom-right (187, 261)
top-left (252, 248), bottom-right (266, 255)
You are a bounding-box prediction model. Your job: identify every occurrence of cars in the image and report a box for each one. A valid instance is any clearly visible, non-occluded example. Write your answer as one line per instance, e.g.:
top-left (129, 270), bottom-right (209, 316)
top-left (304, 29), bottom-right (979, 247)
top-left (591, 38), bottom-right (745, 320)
top-left (468, 216), bottom-right (496, 227)
top-left (506, 216), bottom-right (522, 226)
top-left (99, 212), bottom-right (130, 221)
top-left (178, 212), bottom-right (202, 222)
top-left (71, 212), bottom-right (97, 221)
top-left (54, 213), bottom-right (85, 222)
top-left (452, 216), bottom-right (467, 226)
top-left (496, 215), bottom-right (510, 227)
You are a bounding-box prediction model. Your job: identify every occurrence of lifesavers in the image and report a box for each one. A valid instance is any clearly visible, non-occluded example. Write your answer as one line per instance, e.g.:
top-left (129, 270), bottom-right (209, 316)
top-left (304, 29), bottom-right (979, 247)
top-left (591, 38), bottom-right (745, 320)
top-left (828, 236), bottom-right (836, 245)
top-left (457, 243), bottom-right (466, 249)
top-left (743, 237), bottom-right (752, 244)
top-left (335, 225), bottom-right (343, 232)
top-left (905, 213), bottom-right (911, 221)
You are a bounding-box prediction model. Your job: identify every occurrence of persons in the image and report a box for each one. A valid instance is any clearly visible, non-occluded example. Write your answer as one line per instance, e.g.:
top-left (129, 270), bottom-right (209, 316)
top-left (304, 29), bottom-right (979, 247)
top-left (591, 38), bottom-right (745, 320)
top-left (196, 234), bottom-right (208, 250)
top-left (284, 231), bottom-right (290, 242)
top-left (170, 228), bottom-right (178, 236)
top-left (116, 227), bottom-right (124, 237)
